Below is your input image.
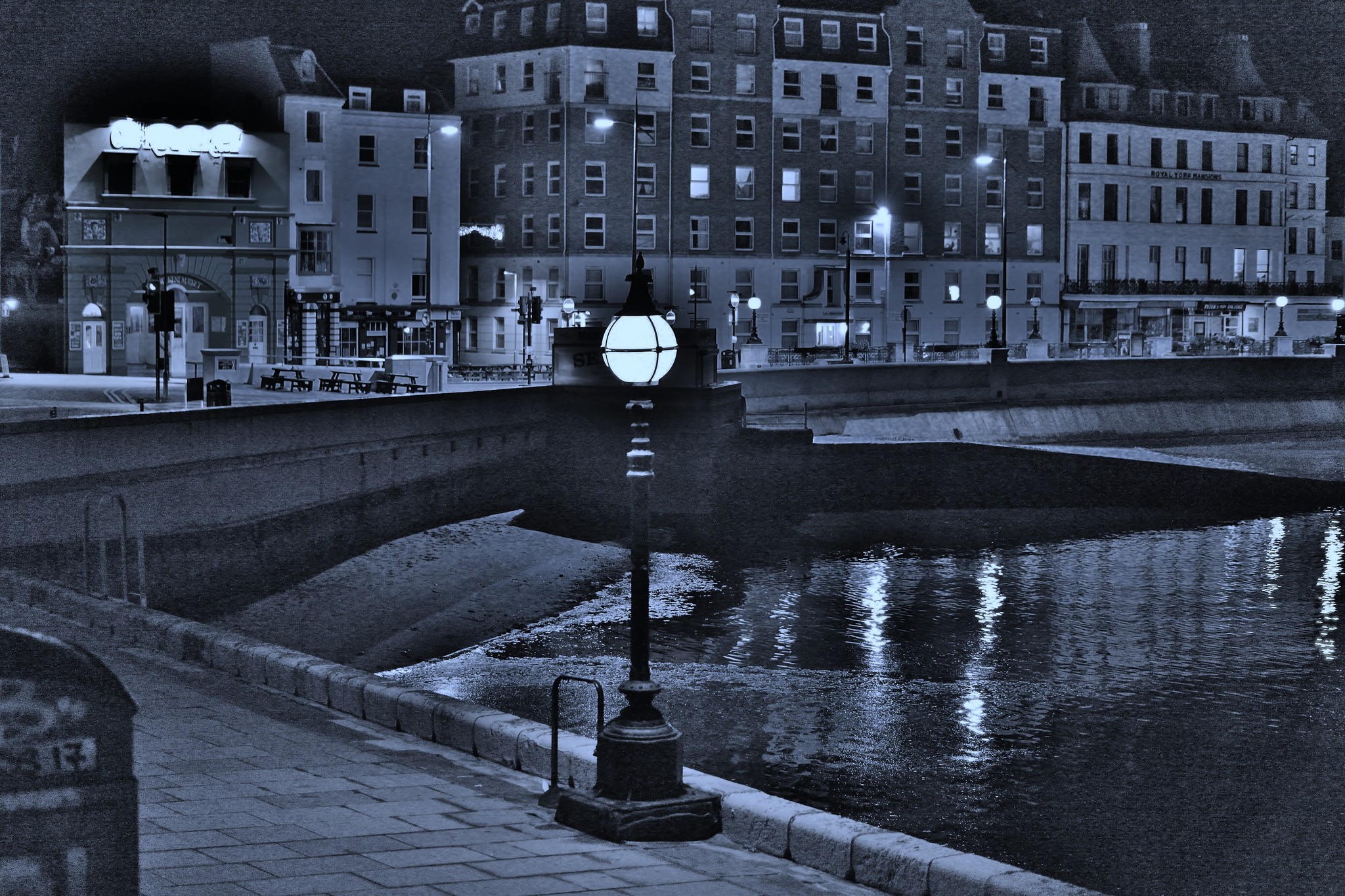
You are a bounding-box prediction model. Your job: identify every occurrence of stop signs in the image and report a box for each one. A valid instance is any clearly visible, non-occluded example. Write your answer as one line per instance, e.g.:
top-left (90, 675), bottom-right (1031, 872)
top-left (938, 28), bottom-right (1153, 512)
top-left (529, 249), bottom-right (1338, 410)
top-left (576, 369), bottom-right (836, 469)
top-left (665, 311), bottom-right (676, 324)
top-left (731, 293), bottom-right (740, 307)
top-left (562, 299), bottom-right (575, 313)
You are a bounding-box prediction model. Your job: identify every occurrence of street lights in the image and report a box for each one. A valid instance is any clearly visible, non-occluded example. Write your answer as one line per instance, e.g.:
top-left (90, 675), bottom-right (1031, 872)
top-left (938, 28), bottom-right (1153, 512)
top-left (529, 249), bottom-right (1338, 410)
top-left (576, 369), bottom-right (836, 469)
top-left (554, 256), bottom-right (722, 843)
top-left (423, 103), bottom-right (457, 356)
top-left (838, 230), bottom-right (855, 363)
top-left (155, 291), bottom-right (175, 331)
top-left (518, 296), bottom-right (528, 316)
top-left (984, 287), bottom-right (1002, 348)
top-left (532, 296), bottom-right (543, 325)
top-left (746, 293), bottom-right (763, 344)
top-left (976, 146), bottom-right (1016, 349)
top-left (594, 94), bottom-right (656, 273)
top-left (1028, 292), bottom-right (1043, 339)
top-left (1330, 294), bottom-right (1345, 344)
top-left (1274, 290), bottom-right (1288, 336)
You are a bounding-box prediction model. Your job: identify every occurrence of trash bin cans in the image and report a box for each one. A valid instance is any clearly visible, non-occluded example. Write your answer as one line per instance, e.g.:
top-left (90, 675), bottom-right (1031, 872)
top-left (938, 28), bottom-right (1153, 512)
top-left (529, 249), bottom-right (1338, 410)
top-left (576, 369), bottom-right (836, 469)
top-left (206, 379), bottom-right (232, 407)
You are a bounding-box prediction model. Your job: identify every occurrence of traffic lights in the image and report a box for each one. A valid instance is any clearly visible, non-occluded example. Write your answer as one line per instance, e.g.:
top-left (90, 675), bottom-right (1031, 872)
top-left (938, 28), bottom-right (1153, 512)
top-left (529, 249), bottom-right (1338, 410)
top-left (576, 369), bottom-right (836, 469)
top-left (147, 279), bottom-right (161, 313)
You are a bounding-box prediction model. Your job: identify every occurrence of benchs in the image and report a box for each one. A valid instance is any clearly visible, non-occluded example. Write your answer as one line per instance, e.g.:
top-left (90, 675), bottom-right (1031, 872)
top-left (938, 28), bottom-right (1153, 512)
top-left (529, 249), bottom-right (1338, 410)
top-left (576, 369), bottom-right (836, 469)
top-left (262, 366), bottom-right (425, 394)
top-left (450, 360), bottom-right (553, 385)
